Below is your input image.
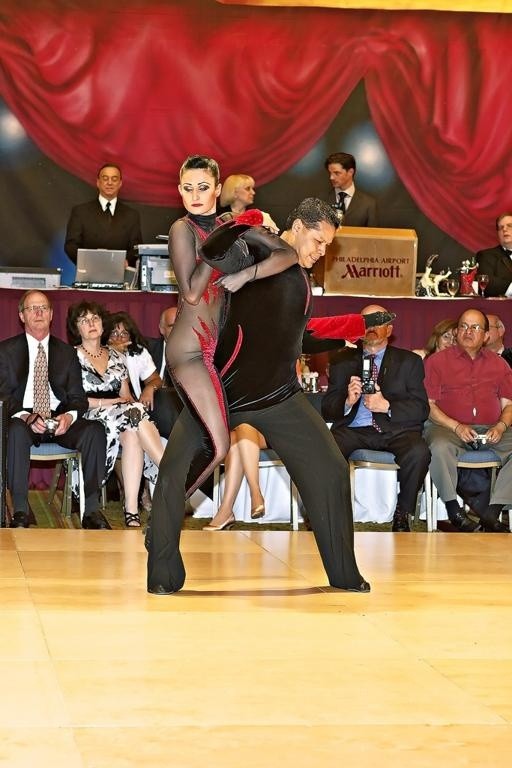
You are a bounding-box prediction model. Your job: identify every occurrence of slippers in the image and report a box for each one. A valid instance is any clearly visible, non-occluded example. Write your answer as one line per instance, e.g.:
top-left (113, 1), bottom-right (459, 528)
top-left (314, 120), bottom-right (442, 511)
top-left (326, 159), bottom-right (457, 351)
top-left (124, 511), bottom-right (141, 526)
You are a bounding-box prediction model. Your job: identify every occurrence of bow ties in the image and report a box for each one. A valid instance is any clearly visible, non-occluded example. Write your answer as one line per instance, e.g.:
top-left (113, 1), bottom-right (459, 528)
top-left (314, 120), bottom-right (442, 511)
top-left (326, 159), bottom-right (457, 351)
top-left (506, 249), bottom-right (512, 255)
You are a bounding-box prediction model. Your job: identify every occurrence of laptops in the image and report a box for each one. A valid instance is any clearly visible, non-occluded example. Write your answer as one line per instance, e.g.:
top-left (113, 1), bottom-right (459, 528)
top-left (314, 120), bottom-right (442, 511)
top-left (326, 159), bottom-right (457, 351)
top-left (73, 248), bottom-right (127, 289)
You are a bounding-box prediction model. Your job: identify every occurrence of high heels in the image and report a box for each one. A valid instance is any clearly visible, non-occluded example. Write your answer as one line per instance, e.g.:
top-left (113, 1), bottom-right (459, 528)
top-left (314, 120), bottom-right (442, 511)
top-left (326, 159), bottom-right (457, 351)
top-left (251, 499), bottom-right (264, 517)
top-left (362, 311), bottom-right (397, 330)
top-left (142, 510), bottom-right (152, 552)
top-left (202, 513), bottom-right (235, 531)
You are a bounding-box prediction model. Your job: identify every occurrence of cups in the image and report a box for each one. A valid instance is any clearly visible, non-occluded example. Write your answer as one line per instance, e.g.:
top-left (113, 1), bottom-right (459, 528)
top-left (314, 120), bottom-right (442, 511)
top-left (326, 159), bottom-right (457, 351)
top-left (475, 434), bottom-right (487, 445)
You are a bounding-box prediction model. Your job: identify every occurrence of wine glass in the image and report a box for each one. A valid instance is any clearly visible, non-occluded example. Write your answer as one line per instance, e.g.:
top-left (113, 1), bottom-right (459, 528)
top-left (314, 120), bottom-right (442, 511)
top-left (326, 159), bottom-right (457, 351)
top-left (477, 274), bottom-right (490, 299)
top-left (446, 279), bottom-right (460, 298)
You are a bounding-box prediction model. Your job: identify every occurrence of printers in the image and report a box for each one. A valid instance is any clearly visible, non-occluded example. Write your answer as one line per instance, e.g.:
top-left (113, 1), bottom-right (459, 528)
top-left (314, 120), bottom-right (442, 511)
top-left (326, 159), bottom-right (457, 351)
top-left (134, 235), bottom-right (179, 292)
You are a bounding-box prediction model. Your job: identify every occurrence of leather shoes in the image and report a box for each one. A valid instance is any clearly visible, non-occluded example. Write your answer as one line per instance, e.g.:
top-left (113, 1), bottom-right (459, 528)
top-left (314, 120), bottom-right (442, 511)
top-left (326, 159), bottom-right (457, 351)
top-left (479, 519), bottom-right (509, 532)
top-left (152, 584), bottom-right (173, 594)
top-left (392, 514), bottom-right (410, 532)
top-left (448, 507), bottom-right (482, 532)
top-left (82, 510), bottom-right (112, 530)
top-left (9, 509), bottom-right (37, 528)
top-left (330, 581), bottom-right (370, 592)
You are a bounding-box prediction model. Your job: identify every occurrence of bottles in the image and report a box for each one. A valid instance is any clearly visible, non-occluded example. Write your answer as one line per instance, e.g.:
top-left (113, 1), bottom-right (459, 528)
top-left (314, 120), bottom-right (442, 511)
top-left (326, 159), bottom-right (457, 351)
top-left (296, 354), bottom-right (320, 394)
top-left (308, 272), bottom-right (317, 287)
top-left (461, 256), bottom-right (479, 296)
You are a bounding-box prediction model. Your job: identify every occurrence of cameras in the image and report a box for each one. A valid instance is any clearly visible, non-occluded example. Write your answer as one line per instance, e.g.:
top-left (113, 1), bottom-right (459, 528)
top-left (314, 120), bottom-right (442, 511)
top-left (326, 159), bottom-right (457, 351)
top-left (359, 359), bottom-right (375, 394)
top-left (473, 434), bottom-right (491, 445)
top-left (43, 417), bottom-right (60, 437)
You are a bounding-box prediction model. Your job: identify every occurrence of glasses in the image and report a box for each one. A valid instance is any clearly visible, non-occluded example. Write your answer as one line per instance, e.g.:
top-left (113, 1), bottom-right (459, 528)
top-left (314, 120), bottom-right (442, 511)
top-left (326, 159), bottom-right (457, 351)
top-left (22, 305), bottom-right (50, 312)
top-left (457, 323), bottom-right (481, 331)
top-left (77, 315), bottom-right (101, 325)
top-left (111, 330), bottom-right (129, 338)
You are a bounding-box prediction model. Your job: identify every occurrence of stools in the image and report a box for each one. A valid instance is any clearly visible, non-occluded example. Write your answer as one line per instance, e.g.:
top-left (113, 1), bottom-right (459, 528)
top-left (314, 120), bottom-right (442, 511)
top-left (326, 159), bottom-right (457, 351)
top-left (212, 446), bottom-right (509, 533)
top-left (5, 442), bottom-right (86, 526)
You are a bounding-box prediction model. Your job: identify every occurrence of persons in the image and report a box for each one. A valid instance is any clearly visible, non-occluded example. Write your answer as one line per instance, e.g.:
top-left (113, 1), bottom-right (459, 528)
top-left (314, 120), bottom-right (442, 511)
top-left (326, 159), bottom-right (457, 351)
top-left (63, 161), bottom-right (144, 274)
top-left (1, 285), bottom-right (221, 532)
top-left (164, 152), bottom-right (302, 501)
top-left (320, 151), bottom-right (379, 232)
top-left (145, 195), bottom-right (368, 595)
top-left (218, 173), bottom-right (279, 231)
top-left (470, 206), bottom-right (511, 298)
top-left (316, 303), bottom-right (511, 532)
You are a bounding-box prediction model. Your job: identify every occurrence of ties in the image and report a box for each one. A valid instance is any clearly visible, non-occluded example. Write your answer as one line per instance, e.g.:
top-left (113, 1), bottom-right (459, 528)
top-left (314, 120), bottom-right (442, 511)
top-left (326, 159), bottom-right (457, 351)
top-left (368, 354), bottom-right (383, 433)
top-left (104, 203), bottom-right (113, 219)
top-left (338, 192), bottom-right (348, 215)
top-left (34, 344), bottom-right (52, 420)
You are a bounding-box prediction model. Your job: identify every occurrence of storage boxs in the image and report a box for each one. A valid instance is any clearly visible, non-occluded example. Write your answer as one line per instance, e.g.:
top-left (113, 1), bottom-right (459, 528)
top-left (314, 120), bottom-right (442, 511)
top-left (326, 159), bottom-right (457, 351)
top-left (312, 226), bottom-right (419, 297)
